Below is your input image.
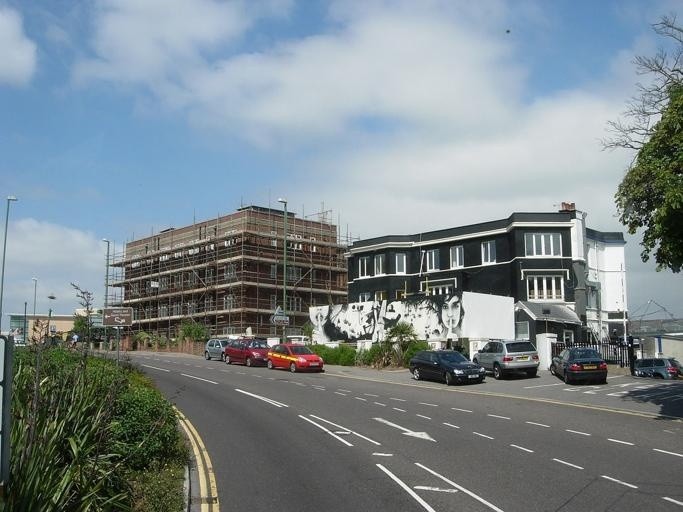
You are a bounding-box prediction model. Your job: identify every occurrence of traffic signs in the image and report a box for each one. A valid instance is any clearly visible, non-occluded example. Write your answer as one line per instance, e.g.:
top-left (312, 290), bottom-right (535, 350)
top-left (101, 307), bottom-right (132, 327)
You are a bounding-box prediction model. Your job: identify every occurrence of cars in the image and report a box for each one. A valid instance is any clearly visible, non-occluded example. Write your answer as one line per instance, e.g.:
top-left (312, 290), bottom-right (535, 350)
top-left (408, 339), bottom-right (540, 386)
top-left (633, 357), bottom-right (683, 380)
top-left (550, 347), bottom-right (608, 384)
top-left (203, 336), bottom-right (324, 373)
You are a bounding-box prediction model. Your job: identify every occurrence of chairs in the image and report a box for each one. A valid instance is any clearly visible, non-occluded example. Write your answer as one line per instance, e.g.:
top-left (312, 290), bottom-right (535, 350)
top-left (234, 341), bottom-right (262, 350)
top-left (571, 350), bottom-right (595, 360)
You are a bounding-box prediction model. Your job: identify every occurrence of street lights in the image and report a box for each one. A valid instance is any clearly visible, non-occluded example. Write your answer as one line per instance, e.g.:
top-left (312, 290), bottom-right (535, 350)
top-left (102, 238), bottom-right (110, 352)
top-left (0, 194), bottom-right (18, 331)
top-left (277, 197), bottom-right (288, 344)
top-left (32, 277), bottom-right (37, 319)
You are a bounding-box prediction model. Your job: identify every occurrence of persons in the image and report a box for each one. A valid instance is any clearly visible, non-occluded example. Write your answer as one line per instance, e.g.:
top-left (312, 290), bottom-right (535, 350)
top-left (437, 290), bottom-right (465, 341)
top-left (308, 304), bottom-right (333, 345)
